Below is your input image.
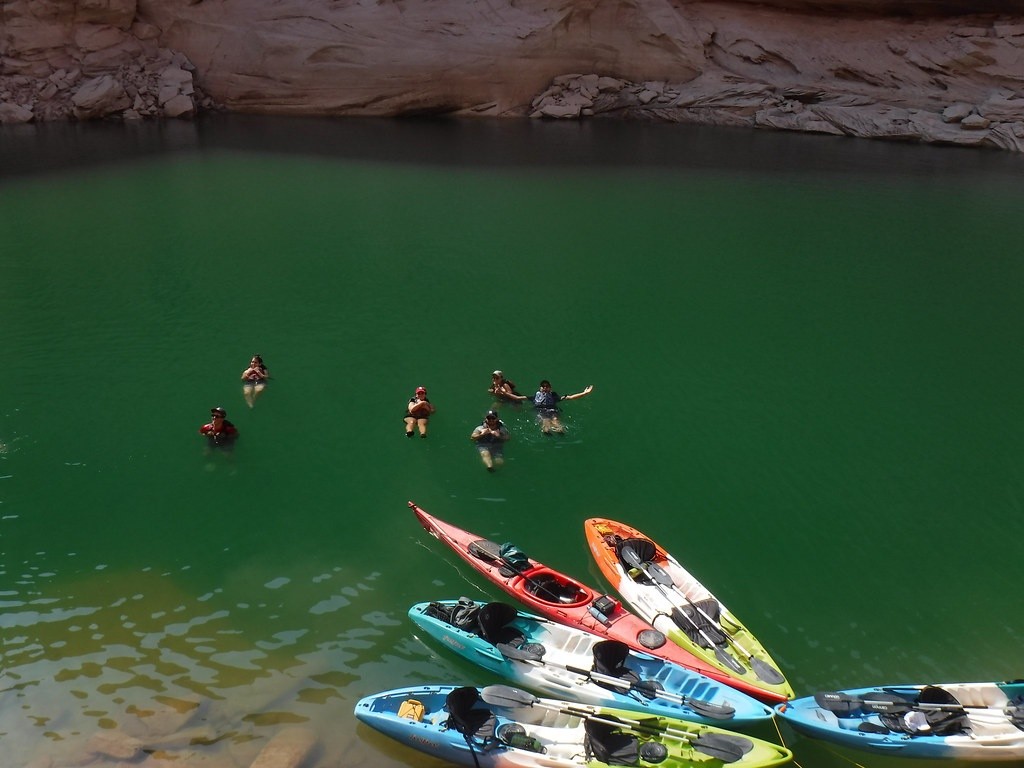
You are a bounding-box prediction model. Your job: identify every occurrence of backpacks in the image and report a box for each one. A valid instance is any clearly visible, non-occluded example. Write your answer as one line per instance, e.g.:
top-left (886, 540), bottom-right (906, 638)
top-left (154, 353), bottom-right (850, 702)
top-left (450, 596), bottom-right (480, 631)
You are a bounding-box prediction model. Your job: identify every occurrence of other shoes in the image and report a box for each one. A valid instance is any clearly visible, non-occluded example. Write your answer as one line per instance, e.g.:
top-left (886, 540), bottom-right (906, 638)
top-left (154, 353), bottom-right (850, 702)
top-left (544, 432), bottom-right (552, 437)
top-left (487, 467), bottom-right (495, 472)
top-left (557, 430), bottom-right (564, 436)
top-left (420, 433), bottom-right (427, 438)
top-left (406, 430), bottom-right (414, 437)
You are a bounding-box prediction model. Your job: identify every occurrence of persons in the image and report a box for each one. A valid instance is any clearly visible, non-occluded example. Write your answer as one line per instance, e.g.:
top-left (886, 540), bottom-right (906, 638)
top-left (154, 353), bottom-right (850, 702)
top-left (499, 380), bottom-right (593, 405)
top-left (402, 386), bottom-right (431, 412)
top-left (470, 410), bottom-right (510, 441)
top-left (199, 408), bottom-right (240, 446)
top-left (488, 370), bottom-right (521, 396)
top-left (241, 355), bottom-right (269, 379)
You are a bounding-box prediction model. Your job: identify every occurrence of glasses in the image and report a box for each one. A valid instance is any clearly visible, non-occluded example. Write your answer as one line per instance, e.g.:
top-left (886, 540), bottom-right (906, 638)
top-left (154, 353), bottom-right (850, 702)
top-left (492, 376), bottom-right (499, 379)
top-left (487, 416), bottom-right (496, 420)
top-left (212, 414), bottom-right (223, 419)
top-left (541, 385), bottom-right (549, 388)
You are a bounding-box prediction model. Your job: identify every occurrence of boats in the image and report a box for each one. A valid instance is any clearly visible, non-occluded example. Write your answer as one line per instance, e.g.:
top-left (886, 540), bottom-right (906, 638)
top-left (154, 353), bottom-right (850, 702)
top-left (583, 514), bottom-right (796, 702)
top-left (352, 684), bottom-right (798, 768)
top-left (407, 500), bottom-right (792, 706)
top-left (772, 681), bottom-right (1024, 768)
top-left (407, 596), bottom-right (779, 732)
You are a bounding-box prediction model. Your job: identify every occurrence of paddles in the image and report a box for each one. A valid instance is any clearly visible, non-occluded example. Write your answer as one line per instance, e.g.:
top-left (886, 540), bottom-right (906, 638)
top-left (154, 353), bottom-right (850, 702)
top-left (620, 545), bottom-right (747, 675)
top-left (496, 640), bottom-right (737, 721)
top-left (480, 683), bottom-right (755, 763)
top-left (648, 561), bottom-right (785, 685)
top-left (467, 537), bottom-right (567, 606)
top-left (814, 690), bottom-right (1024, 731)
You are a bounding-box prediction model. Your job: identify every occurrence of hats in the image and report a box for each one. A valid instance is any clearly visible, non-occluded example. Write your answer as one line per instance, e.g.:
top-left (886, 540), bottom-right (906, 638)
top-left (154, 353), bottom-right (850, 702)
top-left (415, 386), bottom-right (426, 393)
top-left (486, 411), bottom-right (498, 418)
top-left (541, 380), bottom-right (551, 388)
top-left (492, 370), bottom-right (503, 378)
top-left (211, 406), bottom-right (226, 418)
